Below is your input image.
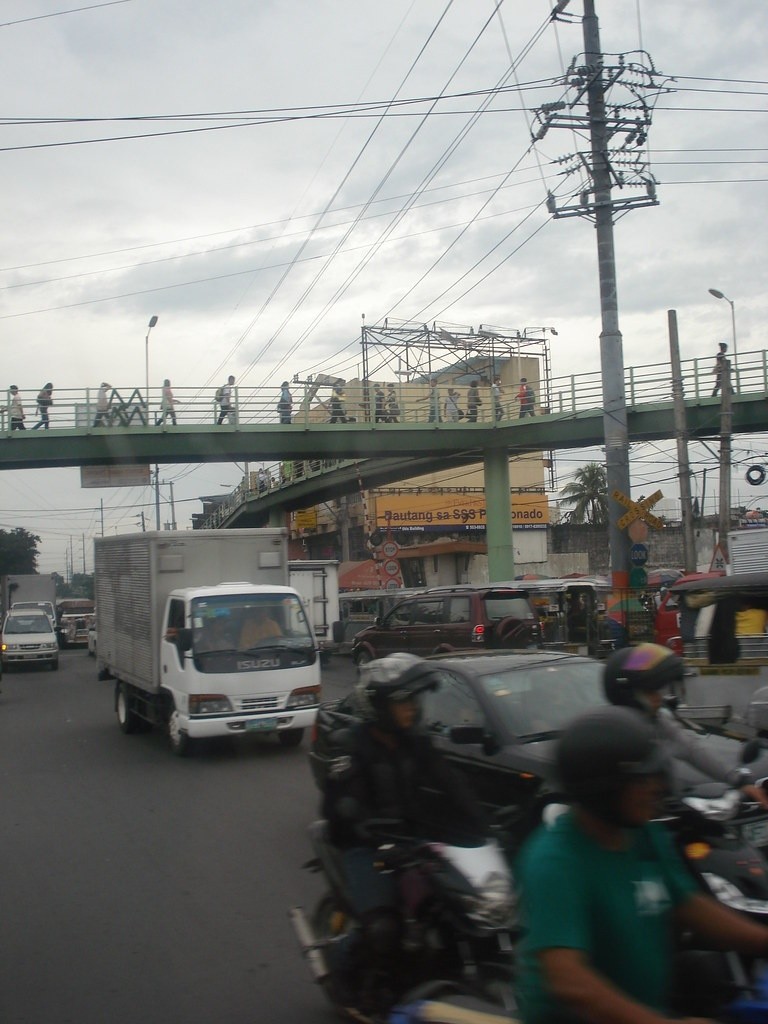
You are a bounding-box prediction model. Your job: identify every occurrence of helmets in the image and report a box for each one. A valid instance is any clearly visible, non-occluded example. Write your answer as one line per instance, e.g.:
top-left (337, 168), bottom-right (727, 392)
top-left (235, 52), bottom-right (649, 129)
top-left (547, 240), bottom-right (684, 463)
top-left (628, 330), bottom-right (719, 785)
top-left (360, 653), bottom-right (445, 704)
top-left (601, 642), bottom-right (685, 716)
top-left (558, 711), bottom-right (666, 822)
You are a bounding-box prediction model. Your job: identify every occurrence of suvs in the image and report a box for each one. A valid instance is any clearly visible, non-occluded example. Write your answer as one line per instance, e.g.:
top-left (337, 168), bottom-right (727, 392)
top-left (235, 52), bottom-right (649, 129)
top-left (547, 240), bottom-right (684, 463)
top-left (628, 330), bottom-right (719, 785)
top-left (351, 584), bottom-right (547, 675)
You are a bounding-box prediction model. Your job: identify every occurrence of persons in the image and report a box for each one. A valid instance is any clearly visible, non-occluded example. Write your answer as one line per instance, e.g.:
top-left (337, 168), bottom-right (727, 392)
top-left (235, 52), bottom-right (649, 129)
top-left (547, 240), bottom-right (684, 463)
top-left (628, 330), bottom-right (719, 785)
top-left (515, 378), bottom-right (535, 419)
top-left (217, 376), bottom-right (235, 425)
top-left (599, 645), bottom-right (768, 806)
top-left (93, 383), bottom-right (112, 426)
top-left (493, 378), bottom-right (504, 421)
top-left (467, 381), bottom-right (480, 422)
top-left (330, 378), bottom-right (347, 423)
top-left (373, 383), bottom-right (398, 423)
top-left (416, 378), bottom-right (443, 423)
top-left (711, 343), bottom-right (734, 396)
top-left (321, 653), bottom-right (481, 1005)
top-left (292, 459), bottom-right (320, 478)
top-left (444, 389), bottom-right (460, 422)
top-left (0, 385), bottom-right (26, 430)
top-left (240, 600), bottom-right (282, 647)
top-left (281, 381), bottom-right (292, 423)
top-left (514, 707), bottom-right (768, 1024)
top-left (258, 463), bottom-right (286, 494)
top-left (32, 383), bottom-right (53, 429)
top-left (157, 379), bottom-right (180, 425)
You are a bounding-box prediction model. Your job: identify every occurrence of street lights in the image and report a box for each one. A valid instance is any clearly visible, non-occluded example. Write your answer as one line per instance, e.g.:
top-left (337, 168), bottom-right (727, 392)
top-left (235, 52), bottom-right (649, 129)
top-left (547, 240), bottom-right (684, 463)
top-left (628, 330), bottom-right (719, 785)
top-left (708, 288), bottom-right (741, 394)
top-left (145, 315), bottom-right (159, 425)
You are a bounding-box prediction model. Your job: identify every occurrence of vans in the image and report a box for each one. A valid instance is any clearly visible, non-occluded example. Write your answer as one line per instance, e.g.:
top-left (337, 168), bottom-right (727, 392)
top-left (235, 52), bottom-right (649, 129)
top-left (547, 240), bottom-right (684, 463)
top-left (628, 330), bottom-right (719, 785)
top-left (54, 595), bottom-right (97, 656)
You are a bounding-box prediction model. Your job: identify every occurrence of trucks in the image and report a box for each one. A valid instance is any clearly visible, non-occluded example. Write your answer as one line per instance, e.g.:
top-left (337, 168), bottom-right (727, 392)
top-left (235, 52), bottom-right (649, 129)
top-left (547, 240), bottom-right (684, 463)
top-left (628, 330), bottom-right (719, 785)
top-left (652, 528), bottom-right (768, 672)
top-left (0, 573), bottom-right (63, 635)
top-left (96, 527), bottom-right (346, 760)
top-left (288, 558), bottom-right (345, 664)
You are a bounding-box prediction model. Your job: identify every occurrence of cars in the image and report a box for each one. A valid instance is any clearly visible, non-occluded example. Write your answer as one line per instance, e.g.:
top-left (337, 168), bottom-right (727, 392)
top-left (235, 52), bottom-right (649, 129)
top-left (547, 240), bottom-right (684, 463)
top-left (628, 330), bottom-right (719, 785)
top-left (308, 646), bottom-right (768, 871)
top-left (0, 606), bottom-right (63, 672)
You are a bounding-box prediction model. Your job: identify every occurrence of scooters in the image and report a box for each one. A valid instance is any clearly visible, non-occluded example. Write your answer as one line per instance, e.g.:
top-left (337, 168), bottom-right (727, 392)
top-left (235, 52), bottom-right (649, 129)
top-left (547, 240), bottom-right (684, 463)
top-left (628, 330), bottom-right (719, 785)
top-left (279, 738), bottom-right (767, 1024)
